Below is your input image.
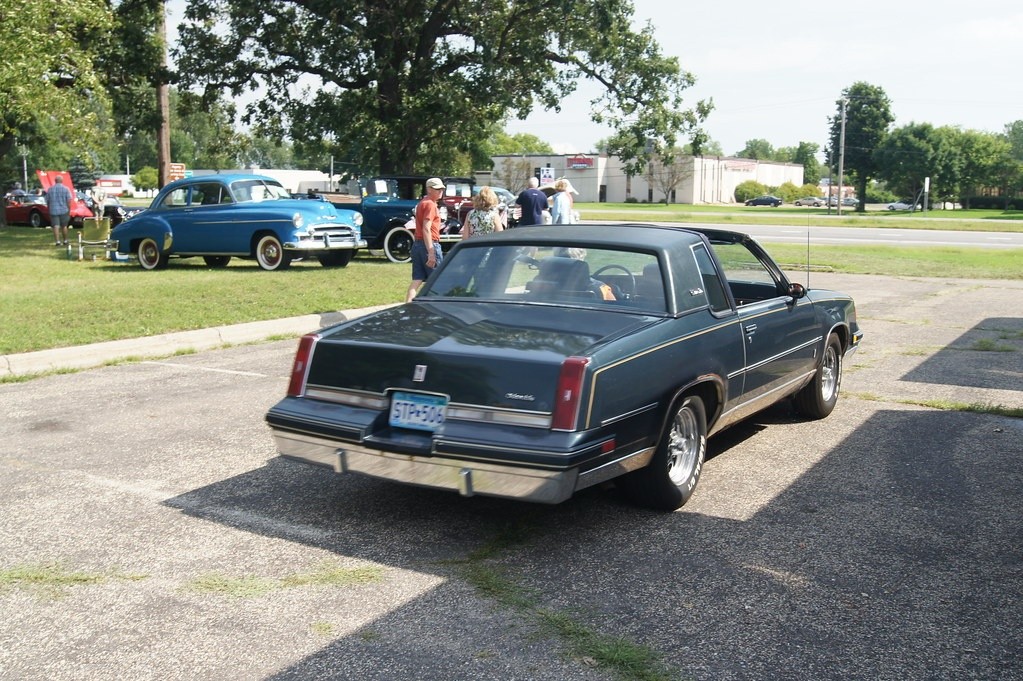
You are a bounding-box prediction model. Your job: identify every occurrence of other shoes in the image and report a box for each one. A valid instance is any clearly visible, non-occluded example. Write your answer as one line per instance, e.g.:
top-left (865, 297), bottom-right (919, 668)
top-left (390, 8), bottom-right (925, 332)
top-left (56, 243), bottom-right (62, 246)
top-left (64, 241), bottom-right (69, 245)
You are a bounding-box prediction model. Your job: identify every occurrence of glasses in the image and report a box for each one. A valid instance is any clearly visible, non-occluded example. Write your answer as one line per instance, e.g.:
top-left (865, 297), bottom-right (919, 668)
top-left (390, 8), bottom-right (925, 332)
top-left (430, 187), bottom-right (445, 191)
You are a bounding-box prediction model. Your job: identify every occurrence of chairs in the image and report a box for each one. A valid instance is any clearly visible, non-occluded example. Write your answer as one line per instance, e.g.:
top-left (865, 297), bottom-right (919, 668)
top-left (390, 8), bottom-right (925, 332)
top-left (637, 264), bottom-right (666, 310)
top-left (77, 216), bottom-right (112, 260)
top-left (533, 257), bottom-right (604, 301)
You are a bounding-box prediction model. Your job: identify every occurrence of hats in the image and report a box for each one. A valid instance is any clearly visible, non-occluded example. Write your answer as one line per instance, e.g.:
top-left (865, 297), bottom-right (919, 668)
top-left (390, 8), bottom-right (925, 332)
top-left (426, 179), bottom-right (446, 189)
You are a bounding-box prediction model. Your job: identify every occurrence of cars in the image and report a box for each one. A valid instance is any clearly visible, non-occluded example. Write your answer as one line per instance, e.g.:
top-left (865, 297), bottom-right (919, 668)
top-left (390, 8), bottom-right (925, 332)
top-left (364, 172), bottom-right (503, 242)
top-left (468, 182), bottom-right (554, 233)
top-left (264, 224), bottom-right (863, 515)
top-left (745, 195), bottom-right (783, 208)
top-left (823, 196), bottom-right (840, 207)
top-left (795, 197), bottom-right (824, 207)
top-left (290, 184), bottom-right (454, 263)
top-left (3, 193), bottom-right (95, 228)
top-left (76, 191), bottom-right (146, 227)
top-left (842, 197), bottom-right (860, 206)
top-left (887, 198), bottom-right (922, 210)
top-left (109, 172), bottom-right (369, 271)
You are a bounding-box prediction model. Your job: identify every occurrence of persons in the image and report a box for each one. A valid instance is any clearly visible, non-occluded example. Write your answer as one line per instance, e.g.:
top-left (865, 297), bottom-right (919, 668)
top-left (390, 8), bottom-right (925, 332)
top-left (3, 180), bottom-right (47, 206)
top-left (89, 178), bottom-right (108, 229)
top-left (513, 174), bottom-right (580, 227)
top-left (551, 244), bottom-right (617, 301)
top-left (405, 177), bottom-right (448, 304)
top-left (44, 174), bottom-right (73, 245)
top-left (463, 185), bottom-right (504, 241)
